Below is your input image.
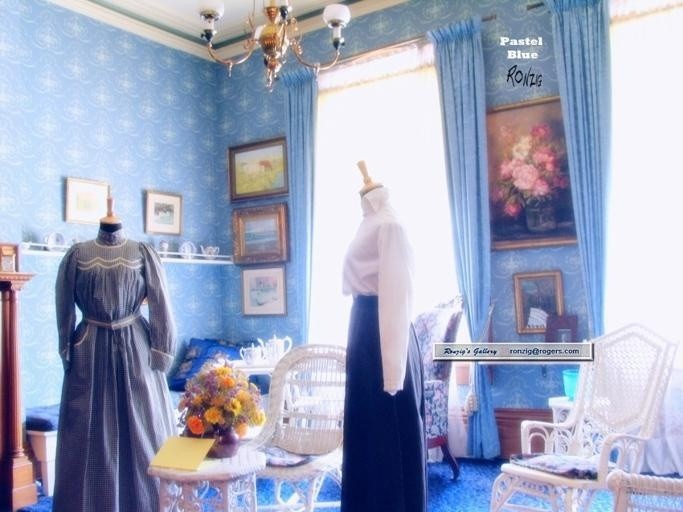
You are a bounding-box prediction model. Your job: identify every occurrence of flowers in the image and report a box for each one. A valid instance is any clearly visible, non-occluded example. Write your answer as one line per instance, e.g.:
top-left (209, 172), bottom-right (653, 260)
top-left (174, 352), bottom-right (265, 439)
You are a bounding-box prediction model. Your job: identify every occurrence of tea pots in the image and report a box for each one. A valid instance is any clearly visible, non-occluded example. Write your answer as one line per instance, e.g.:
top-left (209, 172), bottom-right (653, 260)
top-left (239, 334), bottom-right (292, 367)
top-left (199, 244), bottom-right (219, 260)
top-left (177, 240), bottom-right (197, 260)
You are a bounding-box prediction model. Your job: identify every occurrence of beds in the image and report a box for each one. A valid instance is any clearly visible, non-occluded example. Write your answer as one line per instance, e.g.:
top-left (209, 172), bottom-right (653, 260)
top-left (220, 355), bottom-right (310, 449)
top-left (25, 335), bottom-right (271, 498)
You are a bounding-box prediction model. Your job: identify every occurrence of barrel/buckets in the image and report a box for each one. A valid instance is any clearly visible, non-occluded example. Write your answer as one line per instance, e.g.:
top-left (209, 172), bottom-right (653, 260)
top-left (563, 369), bottom-right (580, 402)
top-left (522, 201), bottom-right (559, 235)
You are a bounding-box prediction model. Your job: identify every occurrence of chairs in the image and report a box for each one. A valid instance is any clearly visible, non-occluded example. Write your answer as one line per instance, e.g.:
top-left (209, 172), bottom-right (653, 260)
top-left (413, 291), bottom-right (464, 482)
top-left (244, 345), bottom-right (346, 511)
top-left (490, 321), bottom-right (683, 512)
top-left (603, 466), bottom-right (683, 512)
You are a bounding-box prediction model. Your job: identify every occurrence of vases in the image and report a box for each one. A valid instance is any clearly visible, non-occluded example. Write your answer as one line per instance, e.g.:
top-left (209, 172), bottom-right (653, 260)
top-left (204, 425), bottom-right (238, 456)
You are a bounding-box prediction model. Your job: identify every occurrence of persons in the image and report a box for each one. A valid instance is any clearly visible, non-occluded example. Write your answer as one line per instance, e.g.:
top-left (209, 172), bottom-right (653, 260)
top-left (340, 186), bottom-right (427, 512)
top-left (52, 217), bottom-right (179, 512)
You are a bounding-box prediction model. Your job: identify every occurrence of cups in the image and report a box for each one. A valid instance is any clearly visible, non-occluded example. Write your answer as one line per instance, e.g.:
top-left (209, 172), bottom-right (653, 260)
top-left (561, 368), bottom-right (578, 401)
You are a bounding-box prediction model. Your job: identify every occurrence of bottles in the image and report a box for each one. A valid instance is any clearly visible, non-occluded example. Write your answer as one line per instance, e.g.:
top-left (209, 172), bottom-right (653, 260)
top-left (159, 239), bottom-right (168, 256)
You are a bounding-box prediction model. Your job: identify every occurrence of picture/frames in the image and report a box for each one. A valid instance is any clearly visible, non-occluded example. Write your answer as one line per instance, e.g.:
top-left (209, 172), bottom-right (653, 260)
top-left (240, 265), bottom-right (287, 317)
top-left (485, 96), bottom-right (579, 251)
top-left (63, 176), bottom-right (113, 225)
top-left (511, 270), bottom-right (567, 335)
top-left (142, 189), bottom-right (184, 236)
top-left (232, 202), bottom-right (288, 266)
top-left (227, 136), bottom-right (289, 201)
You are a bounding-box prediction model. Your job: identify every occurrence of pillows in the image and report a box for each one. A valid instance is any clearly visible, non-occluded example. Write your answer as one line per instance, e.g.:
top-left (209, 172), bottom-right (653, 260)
top-left (166, 337), bottom-right (277, 396)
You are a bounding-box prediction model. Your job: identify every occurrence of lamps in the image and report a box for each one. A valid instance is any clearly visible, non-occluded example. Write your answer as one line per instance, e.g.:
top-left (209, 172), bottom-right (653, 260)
top-left (197, 0), bottom-right (352, 94)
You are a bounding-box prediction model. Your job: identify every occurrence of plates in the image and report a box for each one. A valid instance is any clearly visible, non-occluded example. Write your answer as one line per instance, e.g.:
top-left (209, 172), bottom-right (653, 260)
top-left (46, 233), bottom-right (65, 253)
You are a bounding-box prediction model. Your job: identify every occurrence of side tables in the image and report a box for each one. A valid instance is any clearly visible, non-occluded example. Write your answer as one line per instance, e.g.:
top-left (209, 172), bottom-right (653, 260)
top-left (148, 446), bottom-right (266, 511)
top-left (548, 395), bottom-right (610, 453)
